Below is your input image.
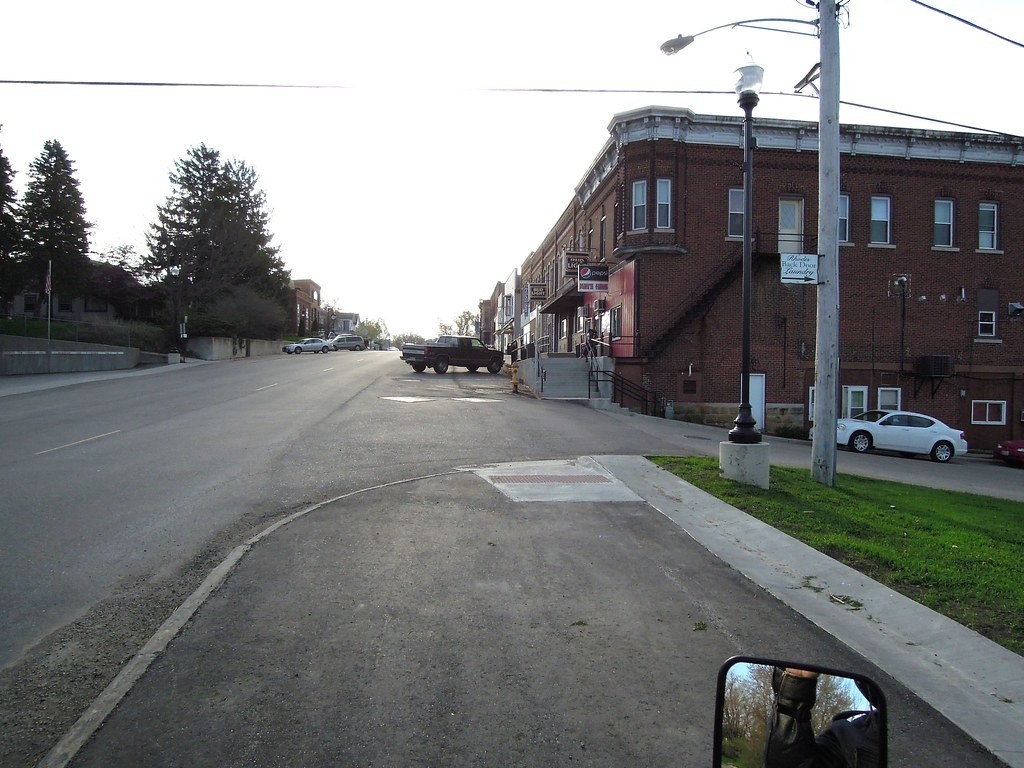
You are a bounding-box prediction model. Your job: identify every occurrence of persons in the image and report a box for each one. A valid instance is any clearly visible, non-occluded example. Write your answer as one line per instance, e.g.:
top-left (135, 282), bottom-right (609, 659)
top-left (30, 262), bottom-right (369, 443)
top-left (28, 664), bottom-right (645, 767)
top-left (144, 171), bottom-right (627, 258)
top-left (765, 665), bottom-right (886, 768)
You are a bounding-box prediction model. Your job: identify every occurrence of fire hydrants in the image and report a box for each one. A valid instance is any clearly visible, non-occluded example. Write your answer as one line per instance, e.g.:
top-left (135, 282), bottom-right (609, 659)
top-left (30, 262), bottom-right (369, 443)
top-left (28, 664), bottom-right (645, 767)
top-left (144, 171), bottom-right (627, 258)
top-left (511, 361), bottom-right (520, 393)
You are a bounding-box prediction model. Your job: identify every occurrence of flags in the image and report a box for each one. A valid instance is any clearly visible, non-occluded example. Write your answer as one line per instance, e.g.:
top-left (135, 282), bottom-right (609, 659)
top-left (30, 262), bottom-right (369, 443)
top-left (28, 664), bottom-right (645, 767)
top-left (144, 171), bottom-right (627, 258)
top-left (45, 265), bottom-right (51, 293)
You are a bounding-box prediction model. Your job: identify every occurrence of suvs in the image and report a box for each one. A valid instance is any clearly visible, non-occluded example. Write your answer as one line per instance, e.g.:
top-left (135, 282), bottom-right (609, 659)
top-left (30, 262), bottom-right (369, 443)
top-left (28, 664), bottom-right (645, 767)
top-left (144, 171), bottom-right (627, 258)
top-left (329, 334), bottom-right (366, 351)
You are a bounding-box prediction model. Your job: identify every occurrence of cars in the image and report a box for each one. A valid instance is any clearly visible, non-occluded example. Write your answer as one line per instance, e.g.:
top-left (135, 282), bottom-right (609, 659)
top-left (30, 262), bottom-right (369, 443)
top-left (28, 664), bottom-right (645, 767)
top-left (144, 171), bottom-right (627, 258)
top-left (995, 439), bottom-right (1024, 466)
top-left (809, 409), bottom-right (969, 462)
top-left (282, 338), bottom-right (332, 355)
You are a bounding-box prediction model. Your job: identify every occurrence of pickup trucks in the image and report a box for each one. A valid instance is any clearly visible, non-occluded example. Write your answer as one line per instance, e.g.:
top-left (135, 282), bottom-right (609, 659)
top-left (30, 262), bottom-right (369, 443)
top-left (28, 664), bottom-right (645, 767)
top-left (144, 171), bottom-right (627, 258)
top-left (400, 336), bottom-right (505, 374)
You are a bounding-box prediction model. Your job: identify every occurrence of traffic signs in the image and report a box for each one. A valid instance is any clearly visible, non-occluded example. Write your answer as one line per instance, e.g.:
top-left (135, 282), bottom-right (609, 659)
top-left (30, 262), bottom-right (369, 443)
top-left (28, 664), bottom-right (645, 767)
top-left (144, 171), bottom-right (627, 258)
top-left (781, 252), bottom-right (817, 285)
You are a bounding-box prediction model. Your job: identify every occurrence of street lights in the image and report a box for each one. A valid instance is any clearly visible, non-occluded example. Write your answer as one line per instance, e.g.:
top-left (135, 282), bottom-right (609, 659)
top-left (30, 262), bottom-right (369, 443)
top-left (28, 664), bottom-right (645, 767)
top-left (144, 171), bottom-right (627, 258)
top-left (659, 17), bottom-right (840, 488)
top-left (728, 46), bottom-right (767, 443)
